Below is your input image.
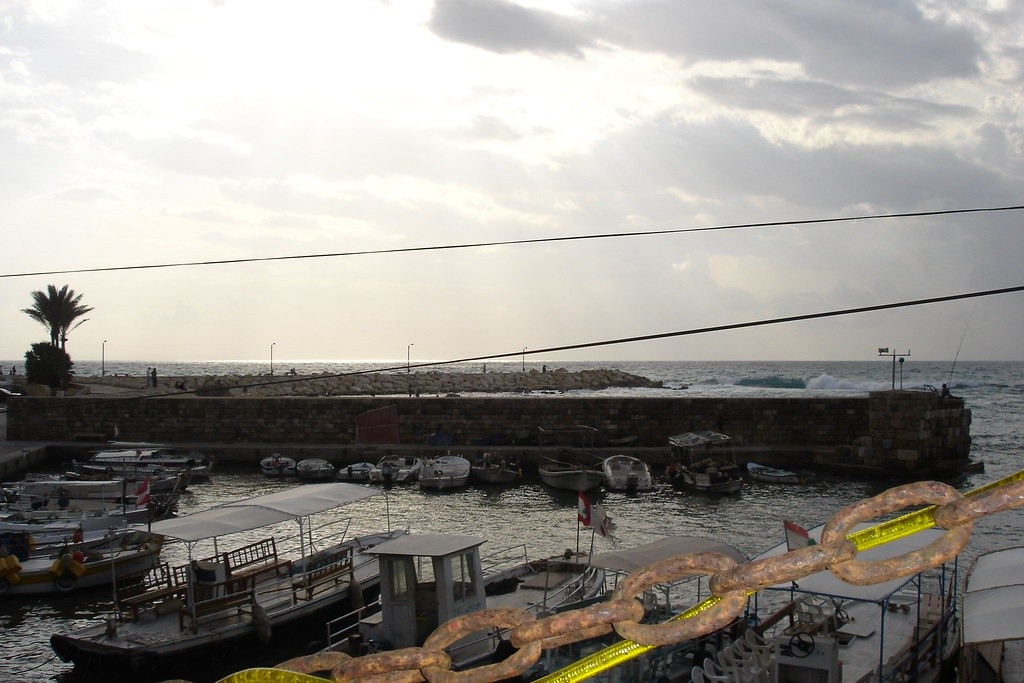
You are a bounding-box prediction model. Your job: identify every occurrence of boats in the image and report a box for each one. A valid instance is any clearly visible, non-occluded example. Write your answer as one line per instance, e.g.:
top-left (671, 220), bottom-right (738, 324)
top-left (49, 483), bottom-right (417, 683)
top-left (747, 459), bottom-right (805, 486)
top-left (667, 428), bottom-right (745, 495)
top-left (959, 544), bottom-right (1024, 683)
top-left (0, 435), bottom-right (217, 597)
top-left (417, 450), bottom-right (470, 490)
top-left (313, 530), bottom-right (606, 671)
top-left (537, 422), bottom-right (606, 492)
top-left (509, 534), bottom-right (767, 682)
top-left (259, 453), bottom-right (297, 478)
top-left (600, 453), bottom-right (655, 494)
top-left (689, 519), bottom-right (962, 683)
top-left (295, 457), bottom-right (334, 481)
top-left (333, 460), bottom-right (373, 483)
top-left (465, 449), bottom-right (523, 485)
top-left (367, 455), bottom-right (424, 484)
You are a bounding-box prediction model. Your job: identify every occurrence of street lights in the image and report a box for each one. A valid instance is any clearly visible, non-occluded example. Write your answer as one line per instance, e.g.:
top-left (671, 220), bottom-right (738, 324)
top-left (270, 343), bottom-right (277, 375)
top-left (407, 343), bottom-right (414, 374)
top-left (523, 346), bottom-right (527, 373)
top-left (102, 340), bottom-right (107, 377)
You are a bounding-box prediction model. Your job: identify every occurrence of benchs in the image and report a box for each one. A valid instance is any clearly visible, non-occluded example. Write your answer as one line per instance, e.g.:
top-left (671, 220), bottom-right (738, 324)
top-left (292, 546), bottom-right (353, 605)
top-left (172, 554), bottom-right (228, 606)
top-left (223, 537), bottom-right (291, 594)
top-left (179, 573), bottom-right (256, 634)
top-left (784, 620), bottom-right (818, 636)
top-left (116, 562), bottom-right (187, 623)
top-left (877, 611), bottom-right (952, 683)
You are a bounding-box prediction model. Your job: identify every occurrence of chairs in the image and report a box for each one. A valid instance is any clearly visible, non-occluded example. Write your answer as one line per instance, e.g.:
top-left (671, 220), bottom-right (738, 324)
top-left (691, 629), bottom-right (776, 683)
top-left (795, 593), bottom-right (837, 636)
top-left (921, 593), bottom-right (947, 619)
top-left (642, 590), bottom-right (667, 624)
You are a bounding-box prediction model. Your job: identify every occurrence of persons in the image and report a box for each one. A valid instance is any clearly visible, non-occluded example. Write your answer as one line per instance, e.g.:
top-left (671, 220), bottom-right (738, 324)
top-left (941, 384), bottom-right (963, 399)
top-left (146, 367), bottom-right (157, 387)
top-left (0, 365), bottom-right (16, 375)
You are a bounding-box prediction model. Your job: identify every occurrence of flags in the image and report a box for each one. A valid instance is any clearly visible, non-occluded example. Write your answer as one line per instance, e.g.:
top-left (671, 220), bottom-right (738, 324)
top-left (134, 474), bottom-right (150, 505)
top-left (577, 490), bottom-right (606, 537)
top-left (784, 518), bottom-right (809, 551)
